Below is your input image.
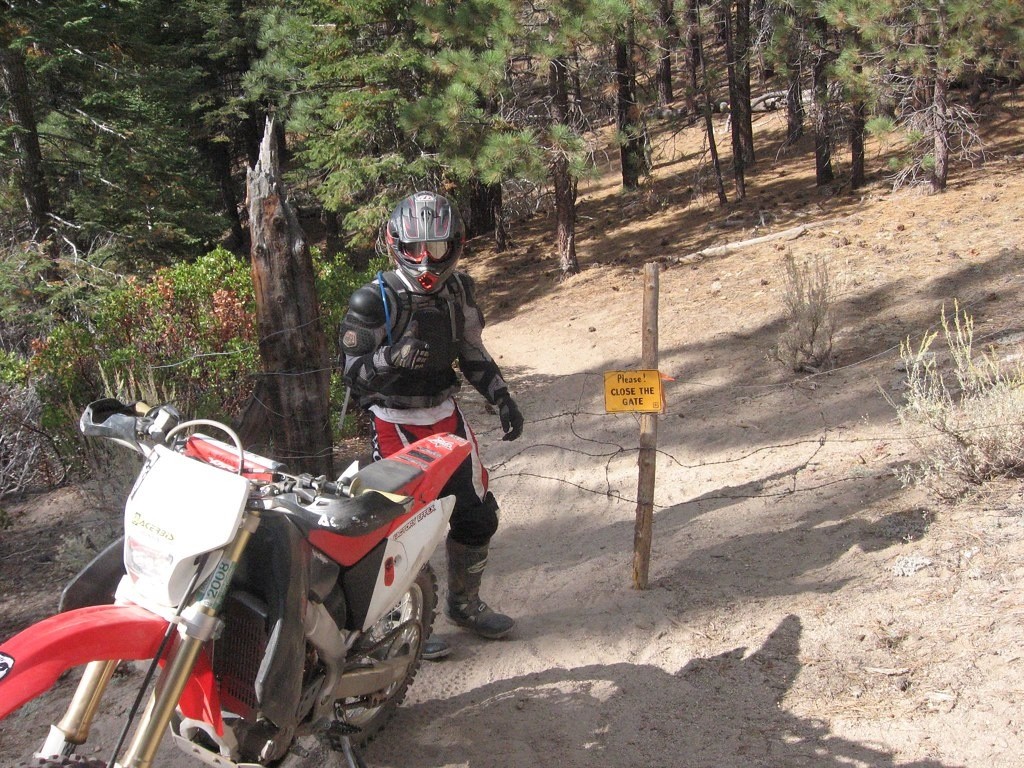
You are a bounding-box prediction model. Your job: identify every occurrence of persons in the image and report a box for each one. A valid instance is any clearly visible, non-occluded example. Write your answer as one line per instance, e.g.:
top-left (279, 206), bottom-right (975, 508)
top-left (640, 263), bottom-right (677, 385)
top-left (337, 191), bottom-right (525, 661)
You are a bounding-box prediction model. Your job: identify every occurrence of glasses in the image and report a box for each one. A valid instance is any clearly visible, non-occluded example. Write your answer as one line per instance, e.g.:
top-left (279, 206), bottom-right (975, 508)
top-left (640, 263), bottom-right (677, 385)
top-left (400, 241), bottom-right (452, 264)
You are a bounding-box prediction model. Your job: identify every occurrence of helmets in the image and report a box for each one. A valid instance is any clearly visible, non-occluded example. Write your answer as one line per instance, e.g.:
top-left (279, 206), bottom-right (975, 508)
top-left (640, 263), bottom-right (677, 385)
top-left (385, 190), bottom-right (465, 295)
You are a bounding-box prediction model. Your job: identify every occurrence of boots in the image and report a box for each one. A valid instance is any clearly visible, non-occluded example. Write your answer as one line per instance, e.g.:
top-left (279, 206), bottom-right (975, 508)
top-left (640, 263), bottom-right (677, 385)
top-left (443, 532), bottom-right (517, 639)
top-left (420, 633), bottom-right (453, 660)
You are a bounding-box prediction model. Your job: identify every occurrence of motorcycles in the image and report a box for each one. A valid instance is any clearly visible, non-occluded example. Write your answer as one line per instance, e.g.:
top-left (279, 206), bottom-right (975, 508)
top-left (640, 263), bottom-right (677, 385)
top-left (1, 393), bottom-right (472, 768)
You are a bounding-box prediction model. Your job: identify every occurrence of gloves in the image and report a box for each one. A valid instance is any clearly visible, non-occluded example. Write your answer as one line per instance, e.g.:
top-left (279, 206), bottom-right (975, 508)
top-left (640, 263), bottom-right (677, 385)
top-left (383, 320), bottom-right (431, 372)
top-left (494, 387), bottom-right (525, 441)
